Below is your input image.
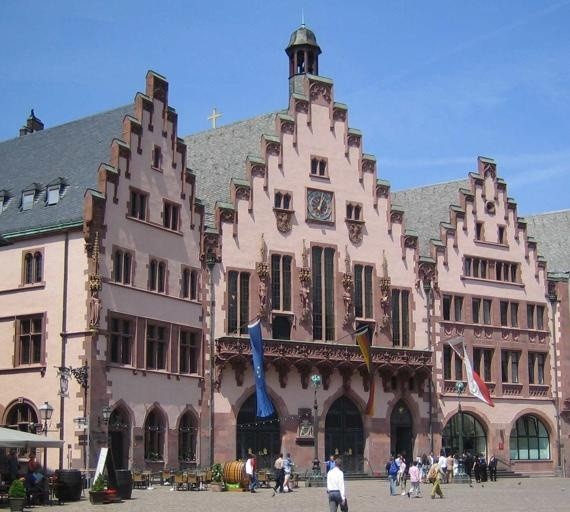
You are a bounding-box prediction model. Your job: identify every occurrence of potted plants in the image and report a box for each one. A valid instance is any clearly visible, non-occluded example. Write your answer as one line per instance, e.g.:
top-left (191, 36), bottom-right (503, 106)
top-left (211, 463), bottom-right (225, 492)
top-left (89, 473), bottom-right (121, 505)
top-left (8, 480), bottom-right (26, 511)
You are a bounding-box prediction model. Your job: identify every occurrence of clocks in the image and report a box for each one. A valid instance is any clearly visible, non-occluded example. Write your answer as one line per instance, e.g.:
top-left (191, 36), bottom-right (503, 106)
top-left (305, 187), bottom-right (336, 222)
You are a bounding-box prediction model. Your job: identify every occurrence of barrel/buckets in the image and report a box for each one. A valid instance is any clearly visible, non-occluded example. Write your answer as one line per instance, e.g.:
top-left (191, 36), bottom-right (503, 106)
top-left (55, 469), bottom-right (83, 503)
top-left (223, 462), bottom-right (250, 491)
top-left (107, 469), bottom-right (132, 499)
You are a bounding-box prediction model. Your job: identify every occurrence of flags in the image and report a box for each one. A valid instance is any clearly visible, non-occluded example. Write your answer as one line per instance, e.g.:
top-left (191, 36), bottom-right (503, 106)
top-left (446, 339), bottom-right (494, 408)
top-left (353, 329), bottom-right (375, 416)
top-left (248, 319), bottom-right (275, 418)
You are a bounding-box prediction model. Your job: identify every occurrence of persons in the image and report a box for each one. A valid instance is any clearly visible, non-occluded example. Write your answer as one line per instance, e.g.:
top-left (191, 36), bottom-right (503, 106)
top-left (327, 459), bottom-right (348, 512)
top-left (246, 454), bottom-right (258, 492)
top-left (382, 448), bottom-right (496, 498)
top-left (280, 453), bottom-right (295, 492)
top-left (323, 455), bottom-right (336, 471)
top-left (272, 453), bottom-right (285, 493)
top-left (9, 448), bottom-right (20, 480)
top-left (28, 453), bottom-right (42, 486)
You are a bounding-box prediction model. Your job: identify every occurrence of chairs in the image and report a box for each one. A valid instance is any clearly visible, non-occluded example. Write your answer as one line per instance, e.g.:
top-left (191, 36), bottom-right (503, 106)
top-left (130, 468), bottom-right (214, 491)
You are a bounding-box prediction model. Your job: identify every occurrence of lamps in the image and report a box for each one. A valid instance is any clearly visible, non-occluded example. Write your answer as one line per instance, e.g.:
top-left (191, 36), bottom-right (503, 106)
top-left (55, 361), bottom-right (89, 398)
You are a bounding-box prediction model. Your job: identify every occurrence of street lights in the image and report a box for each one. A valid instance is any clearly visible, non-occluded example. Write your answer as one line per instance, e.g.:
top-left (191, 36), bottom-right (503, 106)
top-left (453, 379), bottom-right (470, 484)
top-left (307, 373), bottom-right (324, 487)
top-left (39, 402), bottom-right (55, 504)
